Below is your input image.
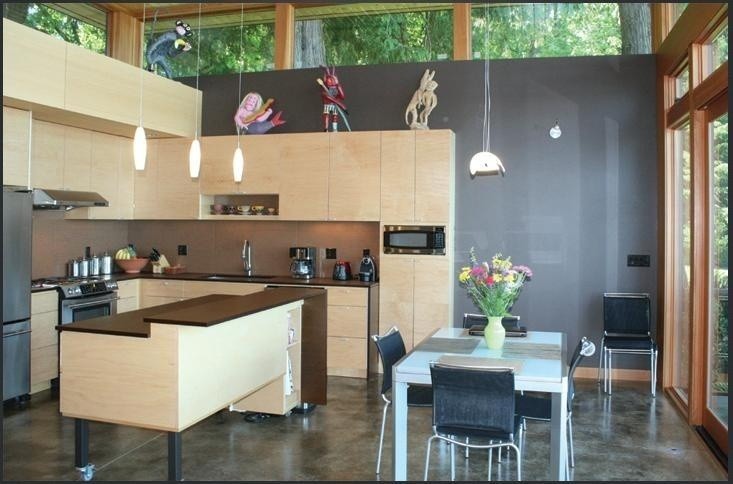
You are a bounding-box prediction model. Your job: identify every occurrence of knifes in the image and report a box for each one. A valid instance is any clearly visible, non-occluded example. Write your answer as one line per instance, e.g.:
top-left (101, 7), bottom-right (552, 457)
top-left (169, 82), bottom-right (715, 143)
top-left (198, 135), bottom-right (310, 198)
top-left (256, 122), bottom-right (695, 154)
top-left (148, 246), bottom-right (168, 274)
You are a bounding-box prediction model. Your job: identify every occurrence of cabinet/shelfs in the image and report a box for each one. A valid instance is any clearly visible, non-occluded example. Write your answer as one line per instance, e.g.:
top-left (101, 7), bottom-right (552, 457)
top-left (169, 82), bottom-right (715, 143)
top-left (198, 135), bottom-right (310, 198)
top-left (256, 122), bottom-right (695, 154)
top-left (139, 279), bottom-right (265, 310)
top-left (327, 288), bottom-right (369, 379)
top-left (377, 258), bottom-right (449, 374)
top-left (279, 131), bottom-right (382, 221)
top-left (67, 125), bottom-right (135, 222)
top-left (134, 138), bottom-right (199, 221)
top-left (381, 129), bottom-right (456, 226)
top-left (113, 280), bottom-right (138, 314)
top-left (31, 117), bottom-right (91, 194)
top-left (199, 133), bottom-right (278, 220)
top-left (30, 292), bottom-right (62, 396)
top-left (3, 19), bottom-right (202, 138)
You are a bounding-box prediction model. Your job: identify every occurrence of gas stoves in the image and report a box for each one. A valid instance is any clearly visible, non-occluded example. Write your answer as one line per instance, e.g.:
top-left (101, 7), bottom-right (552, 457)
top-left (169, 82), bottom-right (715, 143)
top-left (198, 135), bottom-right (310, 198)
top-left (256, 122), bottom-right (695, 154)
top-left (31, 276), bottom-right (107, 286)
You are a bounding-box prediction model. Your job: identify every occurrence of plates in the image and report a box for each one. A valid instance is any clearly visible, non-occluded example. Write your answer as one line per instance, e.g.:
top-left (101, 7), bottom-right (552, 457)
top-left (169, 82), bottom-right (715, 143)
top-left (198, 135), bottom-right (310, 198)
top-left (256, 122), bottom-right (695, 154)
top-left (238, 212), bottom-right (250, 215)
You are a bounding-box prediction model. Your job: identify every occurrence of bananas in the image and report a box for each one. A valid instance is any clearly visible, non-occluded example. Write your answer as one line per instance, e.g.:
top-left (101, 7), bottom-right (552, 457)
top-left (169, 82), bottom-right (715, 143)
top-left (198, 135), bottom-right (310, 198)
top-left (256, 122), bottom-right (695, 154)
top-left (115, 248), bottom-right (131, 260)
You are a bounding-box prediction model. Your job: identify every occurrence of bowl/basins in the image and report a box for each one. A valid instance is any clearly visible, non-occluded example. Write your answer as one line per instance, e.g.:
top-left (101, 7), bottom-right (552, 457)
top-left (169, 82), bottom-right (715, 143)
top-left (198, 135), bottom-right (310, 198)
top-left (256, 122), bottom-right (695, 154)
top-left (209, 204), bottom-right (237, 215)
top-left (114, 257), bottom-right (151, 274)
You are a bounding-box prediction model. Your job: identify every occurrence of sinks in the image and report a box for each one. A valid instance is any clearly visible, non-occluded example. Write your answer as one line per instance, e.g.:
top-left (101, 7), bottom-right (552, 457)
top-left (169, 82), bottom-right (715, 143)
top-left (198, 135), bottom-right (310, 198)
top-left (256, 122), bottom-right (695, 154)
top-left (209, 274), bottom-right (272, 282)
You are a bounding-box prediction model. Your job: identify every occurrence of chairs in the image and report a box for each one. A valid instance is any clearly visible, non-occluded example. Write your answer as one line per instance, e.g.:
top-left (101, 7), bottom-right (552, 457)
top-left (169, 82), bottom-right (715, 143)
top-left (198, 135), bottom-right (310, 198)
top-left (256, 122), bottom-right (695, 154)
top-left (514, 337), bottom-right (595, 467)
top-left (424, 362), bottom-right (527, 482)
top-left (370, 327), bottom-right (433, 474)
top-left (596, 293), bottom-right (659, 396)
top-left (462, 311), bottom-right (520, 331)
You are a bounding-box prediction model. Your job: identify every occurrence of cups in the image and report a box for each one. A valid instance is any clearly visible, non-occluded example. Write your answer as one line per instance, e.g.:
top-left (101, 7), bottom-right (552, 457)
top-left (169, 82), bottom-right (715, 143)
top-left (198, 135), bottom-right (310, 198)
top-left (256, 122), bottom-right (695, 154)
top-left (251, 206), bottom-right (264, 214)
top-left (237, 205), bottom-right (250, 212)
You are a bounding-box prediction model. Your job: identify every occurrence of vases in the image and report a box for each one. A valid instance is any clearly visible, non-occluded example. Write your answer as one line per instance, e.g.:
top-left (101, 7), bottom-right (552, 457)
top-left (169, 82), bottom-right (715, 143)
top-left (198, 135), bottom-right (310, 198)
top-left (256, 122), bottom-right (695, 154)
top-left (482, 317), bottom-right (507, 352)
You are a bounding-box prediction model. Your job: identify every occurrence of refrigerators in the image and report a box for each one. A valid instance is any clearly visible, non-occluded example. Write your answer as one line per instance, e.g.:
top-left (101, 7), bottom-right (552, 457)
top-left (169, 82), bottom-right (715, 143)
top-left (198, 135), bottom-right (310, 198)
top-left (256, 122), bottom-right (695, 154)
top-left (1, 184), bottom-right (33, 400)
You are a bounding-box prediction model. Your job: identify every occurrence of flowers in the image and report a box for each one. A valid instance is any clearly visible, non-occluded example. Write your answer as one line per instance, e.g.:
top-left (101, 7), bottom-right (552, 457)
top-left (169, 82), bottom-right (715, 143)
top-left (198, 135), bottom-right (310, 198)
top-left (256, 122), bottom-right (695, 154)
top-left (457, 244), bottom-right (533, 317)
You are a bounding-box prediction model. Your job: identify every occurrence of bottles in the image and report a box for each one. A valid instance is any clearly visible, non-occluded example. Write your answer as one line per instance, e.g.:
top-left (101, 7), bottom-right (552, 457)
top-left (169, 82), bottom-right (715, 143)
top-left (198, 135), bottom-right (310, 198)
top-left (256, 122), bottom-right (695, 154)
top-left (67, 251), bottom-right (111, 279)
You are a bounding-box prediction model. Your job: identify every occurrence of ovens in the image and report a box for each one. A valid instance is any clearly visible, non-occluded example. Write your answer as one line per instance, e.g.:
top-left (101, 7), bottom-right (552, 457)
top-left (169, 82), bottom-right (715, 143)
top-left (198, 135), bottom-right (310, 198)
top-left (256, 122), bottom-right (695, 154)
top-left (59, 293), bottom-right (118, 326)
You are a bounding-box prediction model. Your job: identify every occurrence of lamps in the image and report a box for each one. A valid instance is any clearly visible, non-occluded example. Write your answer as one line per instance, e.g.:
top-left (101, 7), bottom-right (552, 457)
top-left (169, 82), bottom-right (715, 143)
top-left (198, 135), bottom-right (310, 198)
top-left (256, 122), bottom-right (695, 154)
top-left (470, 3), bottom-right (509, 176)
top-left (549, 124), bottom-right (562, 139)
top-left (188, 0), bottom-right (201, 175)
top-left (134, 3), bottom-right (149, 169)
top-left (231, 2), bottom-right (245, 182)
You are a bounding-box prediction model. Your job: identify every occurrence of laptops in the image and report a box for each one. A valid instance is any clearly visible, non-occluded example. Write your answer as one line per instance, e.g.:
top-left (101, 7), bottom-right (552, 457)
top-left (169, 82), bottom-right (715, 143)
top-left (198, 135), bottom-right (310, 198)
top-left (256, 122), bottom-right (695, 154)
top-left (470, 324), bottom-right (528, 338)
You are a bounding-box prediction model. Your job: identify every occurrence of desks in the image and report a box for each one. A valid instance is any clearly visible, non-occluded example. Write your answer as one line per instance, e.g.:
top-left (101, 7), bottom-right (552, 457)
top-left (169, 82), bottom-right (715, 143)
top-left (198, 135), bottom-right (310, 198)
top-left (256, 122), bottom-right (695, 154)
top-left (392, 326), bottom-right (569, 481)
top-left (56, 288), bottom-right (327, 483)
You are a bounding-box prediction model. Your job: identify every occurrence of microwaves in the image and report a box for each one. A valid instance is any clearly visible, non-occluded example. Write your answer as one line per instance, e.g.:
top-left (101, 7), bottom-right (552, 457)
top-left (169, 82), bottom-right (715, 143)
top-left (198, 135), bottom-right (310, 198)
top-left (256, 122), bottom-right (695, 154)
top-left (382, 225), bottom-right (447, 255)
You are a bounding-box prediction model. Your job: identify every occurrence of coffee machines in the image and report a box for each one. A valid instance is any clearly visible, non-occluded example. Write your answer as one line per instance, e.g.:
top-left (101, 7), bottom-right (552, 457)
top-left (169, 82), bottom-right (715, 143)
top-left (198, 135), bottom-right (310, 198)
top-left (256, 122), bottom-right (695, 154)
top-left (289, 246), bottom-right (317, 280)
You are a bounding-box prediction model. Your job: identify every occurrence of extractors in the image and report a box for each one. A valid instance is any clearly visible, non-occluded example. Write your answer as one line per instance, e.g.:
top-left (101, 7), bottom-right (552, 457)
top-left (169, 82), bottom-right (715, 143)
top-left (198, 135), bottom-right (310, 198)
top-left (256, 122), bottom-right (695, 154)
top-left (33, 187), bottom-right (110, 211)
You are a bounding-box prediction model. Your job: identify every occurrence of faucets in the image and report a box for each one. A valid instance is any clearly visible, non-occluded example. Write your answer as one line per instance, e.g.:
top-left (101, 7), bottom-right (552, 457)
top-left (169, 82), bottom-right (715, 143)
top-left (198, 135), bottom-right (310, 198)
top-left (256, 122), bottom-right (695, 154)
top-left (240, 239), bottom-right (252, 277)
